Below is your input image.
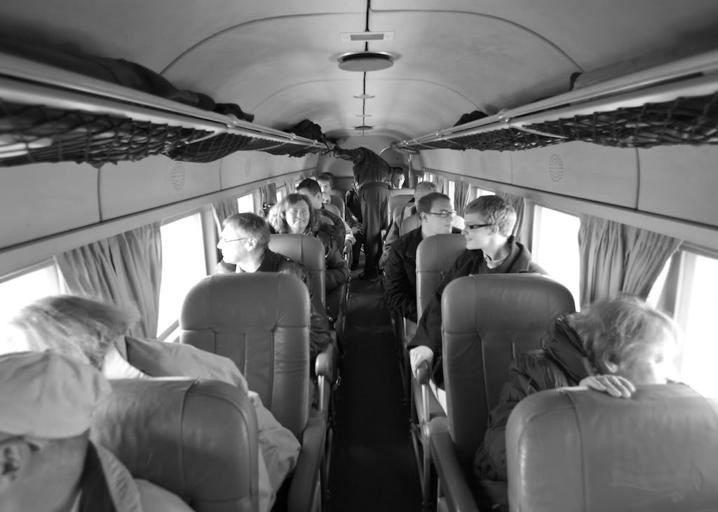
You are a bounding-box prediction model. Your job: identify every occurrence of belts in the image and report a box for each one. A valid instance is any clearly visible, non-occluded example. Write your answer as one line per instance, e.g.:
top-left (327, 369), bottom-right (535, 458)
top-left (359, 182), bottom-right (390, 189)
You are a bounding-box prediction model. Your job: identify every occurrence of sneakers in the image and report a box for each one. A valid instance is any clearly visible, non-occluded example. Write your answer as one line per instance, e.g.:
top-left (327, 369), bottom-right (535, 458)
top-left (359, 272), bottom-right (377, 282)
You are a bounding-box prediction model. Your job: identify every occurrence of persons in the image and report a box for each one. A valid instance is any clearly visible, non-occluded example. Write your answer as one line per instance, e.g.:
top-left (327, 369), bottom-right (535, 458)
top-left (0, 353), bottom-right (195, 512)
top-left (385, 192), bottom-right (468, 320)
top-left (10, 296), bottom-right (301, 510)
top-left (320, 192), bottom-right (357, 254)
top-left (469, 293), bottom-right (675, 510)
top-left (314, 176), bottom-right (332, 193)
top-left (296, 179), bottom-right (345, 256)
top-left (324, 140), bottom-right (395, 277)
top-left (377, 181), bottom-right (439, 276)
top-left (404, 195), bottom-right (555, 414)
top-left (318, 172), bottom-right (336, 189)
top-left (386, 165), bottom-right (411, 190)
top-left (267, 193), bottom-right (352, 292)
top-left (217, 213), bottom-right (332, 365)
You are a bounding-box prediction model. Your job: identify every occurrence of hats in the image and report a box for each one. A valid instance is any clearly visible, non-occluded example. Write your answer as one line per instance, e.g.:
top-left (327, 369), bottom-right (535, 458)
top-left (0, 349), bottom-right (112, 440)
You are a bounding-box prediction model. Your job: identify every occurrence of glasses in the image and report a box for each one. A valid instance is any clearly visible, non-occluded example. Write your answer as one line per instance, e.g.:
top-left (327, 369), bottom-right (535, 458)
top-left (465, 224), bottom-right (493, 230)
top-left (424, 211), bottom-right (456, 218)
top-left (219, 231), bottom-right (251, 244)
